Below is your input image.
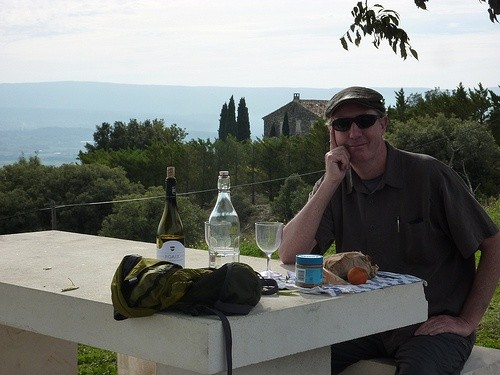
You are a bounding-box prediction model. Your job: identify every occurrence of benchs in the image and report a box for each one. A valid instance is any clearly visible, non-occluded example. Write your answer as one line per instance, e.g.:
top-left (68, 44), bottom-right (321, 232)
top-left (350, 348), bottom-right (500, 375)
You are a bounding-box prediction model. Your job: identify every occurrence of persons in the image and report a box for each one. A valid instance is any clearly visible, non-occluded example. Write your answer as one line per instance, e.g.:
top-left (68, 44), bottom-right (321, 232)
top-left (277, 86), bottom-right (500, 375)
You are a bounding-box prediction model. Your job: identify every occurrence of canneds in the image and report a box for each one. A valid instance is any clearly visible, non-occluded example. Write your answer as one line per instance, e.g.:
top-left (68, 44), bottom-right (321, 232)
top-left (295, 254), bottom-right (323, 288)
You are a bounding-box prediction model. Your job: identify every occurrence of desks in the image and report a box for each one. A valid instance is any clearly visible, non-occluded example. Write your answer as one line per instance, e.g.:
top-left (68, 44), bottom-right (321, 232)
top-left (0, 230), bottom-right (429, 375)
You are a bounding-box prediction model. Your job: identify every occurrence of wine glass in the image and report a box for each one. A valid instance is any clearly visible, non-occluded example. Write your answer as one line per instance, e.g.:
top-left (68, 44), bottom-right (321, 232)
top-left (255, 222), bottom-right (283, 278)
top-left (205, 222), bottom-right (231, 268)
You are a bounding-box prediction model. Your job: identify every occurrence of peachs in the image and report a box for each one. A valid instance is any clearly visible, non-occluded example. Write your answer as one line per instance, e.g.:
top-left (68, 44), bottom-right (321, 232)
top-left (348, 266), bottom-right (369, 285)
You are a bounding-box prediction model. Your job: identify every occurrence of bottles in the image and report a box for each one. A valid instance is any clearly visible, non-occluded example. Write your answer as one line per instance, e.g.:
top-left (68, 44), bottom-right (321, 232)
top-left (157, 167), bottom-right (185, 269)
top-left (209, 171), bottom-right (239, 269)
